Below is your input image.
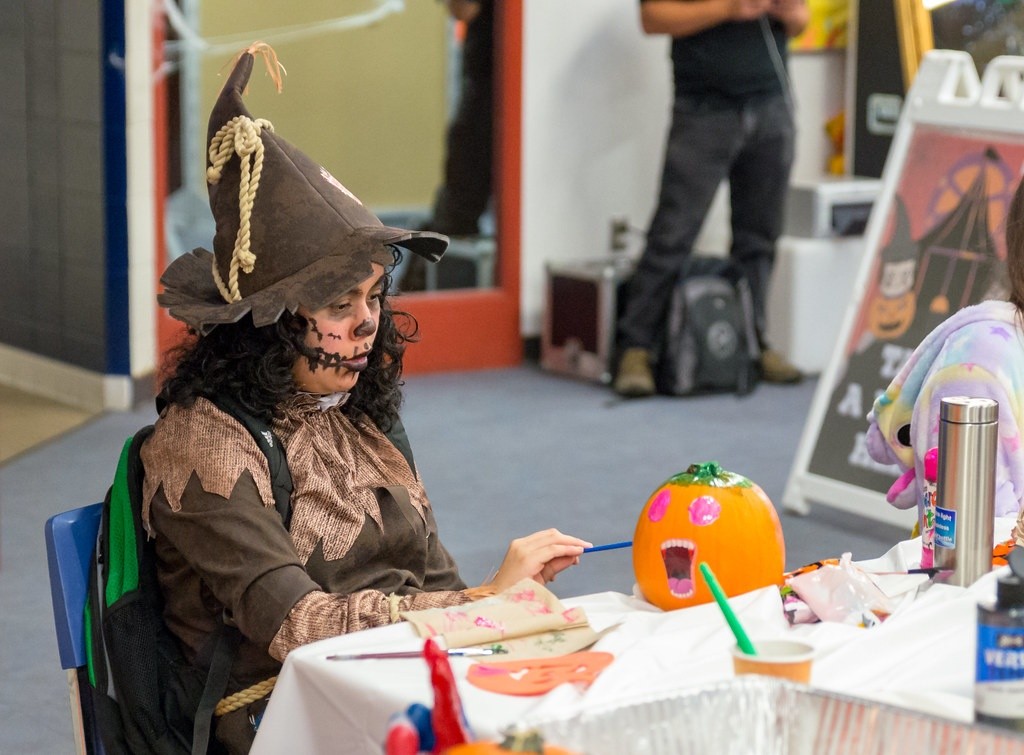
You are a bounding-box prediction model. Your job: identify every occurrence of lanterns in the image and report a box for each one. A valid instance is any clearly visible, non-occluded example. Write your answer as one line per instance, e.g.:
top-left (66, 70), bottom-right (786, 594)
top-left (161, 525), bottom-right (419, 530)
top-left (633, 461), bottom-right (785, 612)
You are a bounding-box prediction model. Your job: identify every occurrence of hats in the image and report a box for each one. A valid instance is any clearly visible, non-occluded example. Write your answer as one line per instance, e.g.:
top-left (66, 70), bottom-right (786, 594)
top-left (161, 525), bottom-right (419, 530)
top-left (156, 40), bottom-right (449, 328)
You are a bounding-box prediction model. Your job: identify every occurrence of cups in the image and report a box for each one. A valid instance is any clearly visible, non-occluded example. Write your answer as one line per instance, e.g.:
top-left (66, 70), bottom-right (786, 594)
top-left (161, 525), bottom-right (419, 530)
top-left (732, 638), bottom-right (815, 684)
top-left (920, 447), bottom-right (938, 569)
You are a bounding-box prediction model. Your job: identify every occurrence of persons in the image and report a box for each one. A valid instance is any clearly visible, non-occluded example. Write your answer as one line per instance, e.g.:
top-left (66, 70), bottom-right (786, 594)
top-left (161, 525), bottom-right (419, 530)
top-left (141, 40), bottom-right (595, 755)
top-left (865, 169), bottom-right (1024, 562)
top-left (398, 0), bottom-right (492, 296)
top-left (609, 0), bottom-right (808, 396)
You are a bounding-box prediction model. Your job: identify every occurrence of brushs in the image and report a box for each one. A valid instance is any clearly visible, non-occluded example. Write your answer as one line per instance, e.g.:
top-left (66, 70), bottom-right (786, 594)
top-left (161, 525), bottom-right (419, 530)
top-left (697, 562), bottom-right (758, 655)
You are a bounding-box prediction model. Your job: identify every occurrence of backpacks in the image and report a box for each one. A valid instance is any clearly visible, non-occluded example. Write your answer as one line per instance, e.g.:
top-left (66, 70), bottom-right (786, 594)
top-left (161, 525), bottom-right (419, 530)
top-left (655, 254), bottom-right (763, 396)
top-left (83, 393), bottom-right (417, 754)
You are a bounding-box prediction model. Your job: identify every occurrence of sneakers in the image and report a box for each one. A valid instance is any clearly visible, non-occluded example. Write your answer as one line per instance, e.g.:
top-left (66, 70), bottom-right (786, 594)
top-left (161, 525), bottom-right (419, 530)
top-left (614, 347), bottom-right (656, 394)
top-left (761, 351), bottom-right (802, 384)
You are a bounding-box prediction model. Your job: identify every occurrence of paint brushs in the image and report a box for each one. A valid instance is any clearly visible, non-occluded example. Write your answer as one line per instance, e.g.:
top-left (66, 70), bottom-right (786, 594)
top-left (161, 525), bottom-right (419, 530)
top-left (581, 541), bottom-right (634, 554)
top-left (327, 650), bottom-right (495, 661)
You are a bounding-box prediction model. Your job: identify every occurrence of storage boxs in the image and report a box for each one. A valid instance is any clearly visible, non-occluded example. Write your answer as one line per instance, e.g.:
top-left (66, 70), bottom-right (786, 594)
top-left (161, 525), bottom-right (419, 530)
top-left (425, 235), bottom-right (496, 292)
top-left (541, 259), bottom-right (627, 386)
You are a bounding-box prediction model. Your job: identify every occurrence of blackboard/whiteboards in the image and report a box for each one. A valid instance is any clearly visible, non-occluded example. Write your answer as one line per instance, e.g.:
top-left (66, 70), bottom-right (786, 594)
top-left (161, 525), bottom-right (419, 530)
top-left (782, 49), bottom-right (1024, 548)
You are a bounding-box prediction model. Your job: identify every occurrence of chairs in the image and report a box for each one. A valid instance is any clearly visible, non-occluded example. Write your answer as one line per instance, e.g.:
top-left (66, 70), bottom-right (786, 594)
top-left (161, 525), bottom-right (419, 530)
top-left (42, 502), bottom-right (127, 754)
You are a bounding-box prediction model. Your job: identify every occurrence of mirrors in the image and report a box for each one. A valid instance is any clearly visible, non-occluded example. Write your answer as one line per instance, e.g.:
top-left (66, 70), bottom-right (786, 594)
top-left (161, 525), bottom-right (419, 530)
top-left (151, 0), bottom-right (523, 381)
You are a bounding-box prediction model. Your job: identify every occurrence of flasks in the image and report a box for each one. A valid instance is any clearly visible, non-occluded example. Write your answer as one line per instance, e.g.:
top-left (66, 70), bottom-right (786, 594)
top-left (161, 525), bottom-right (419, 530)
top-left (933, 397), bottom-right (999, 586)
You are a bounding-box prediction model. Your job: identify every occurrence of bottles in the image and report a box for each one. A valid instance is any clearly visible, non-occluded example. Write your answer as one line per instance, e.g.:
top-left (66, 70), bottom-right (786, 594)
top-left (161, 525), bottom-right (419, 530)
top-left (973, 577), bottom-right (1024, 731)
